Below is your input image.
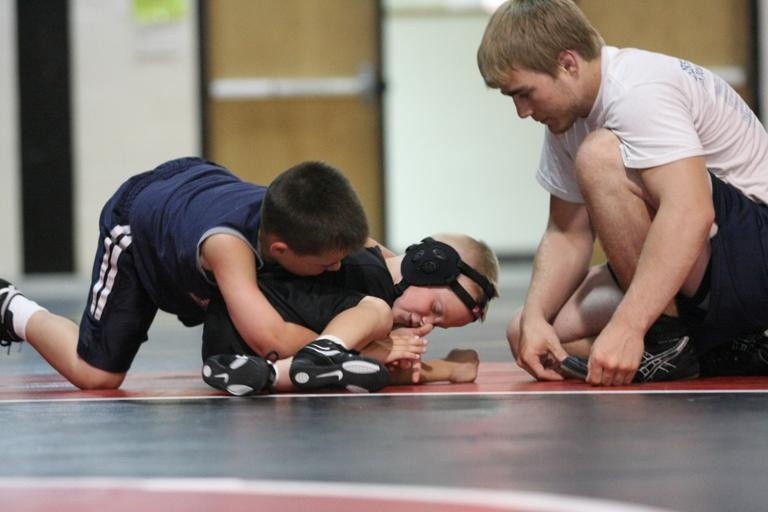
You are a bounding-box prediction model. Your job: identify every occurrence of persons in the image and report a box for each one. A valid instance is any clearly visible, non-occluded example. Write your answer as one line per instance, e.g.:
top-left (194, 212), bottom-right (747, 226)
top-left (200, 231), bottom-right (500, 398)
top-left (475, 0), bottom-right (768, 387)
top-left (0, 149), bottom-right (434, 391)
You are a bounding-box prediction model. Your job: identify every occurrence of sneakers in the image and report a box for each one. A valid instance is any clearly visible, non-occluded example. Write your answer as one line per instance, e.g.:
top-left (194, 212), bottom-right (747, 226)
top-left (289, 339), bottom-right (389, 393)
top-left (633, 316), bottom-right (699, 384)
top-left (560, 356), bottom-right (588, 380)
top-left (202, 353), bottom-right (276, 397)
top-left (0, 279), bottom-right (24, 346)
